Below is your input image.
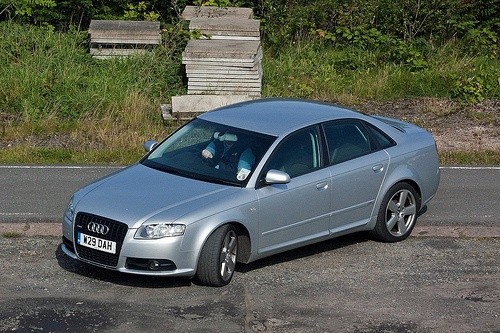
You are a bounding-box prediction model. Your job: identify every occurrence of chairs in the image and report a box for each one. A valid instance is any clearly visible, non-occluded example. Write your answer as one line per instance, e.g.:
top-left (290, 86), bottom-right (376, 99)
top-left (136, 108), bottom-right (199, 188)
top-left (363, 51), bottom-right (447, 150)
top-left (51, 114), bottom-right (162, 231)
top-left (284, 131), bottom-right (317, 176)
top-left (340, 125), bottom-right (368, 161)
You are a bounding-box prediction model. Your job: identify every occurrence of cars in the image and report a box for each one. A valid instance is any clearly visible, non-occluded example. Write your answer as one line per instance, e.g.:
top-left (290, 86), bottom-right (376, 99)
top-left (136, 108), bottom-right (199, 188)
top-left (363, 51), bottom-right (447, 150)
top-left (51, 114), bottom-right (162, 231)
top-left (59, 97), bottom-right (441, 286)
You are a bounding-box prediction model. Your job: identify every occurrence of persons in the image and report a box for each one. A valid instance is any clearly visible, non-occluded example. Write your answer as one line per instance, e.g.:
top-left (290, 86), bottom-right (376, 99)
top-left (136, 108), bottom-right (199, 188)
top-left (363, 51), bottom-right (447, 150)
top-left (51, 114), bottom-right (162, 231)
top-left (201, 128), bottom-right (256, 176)
